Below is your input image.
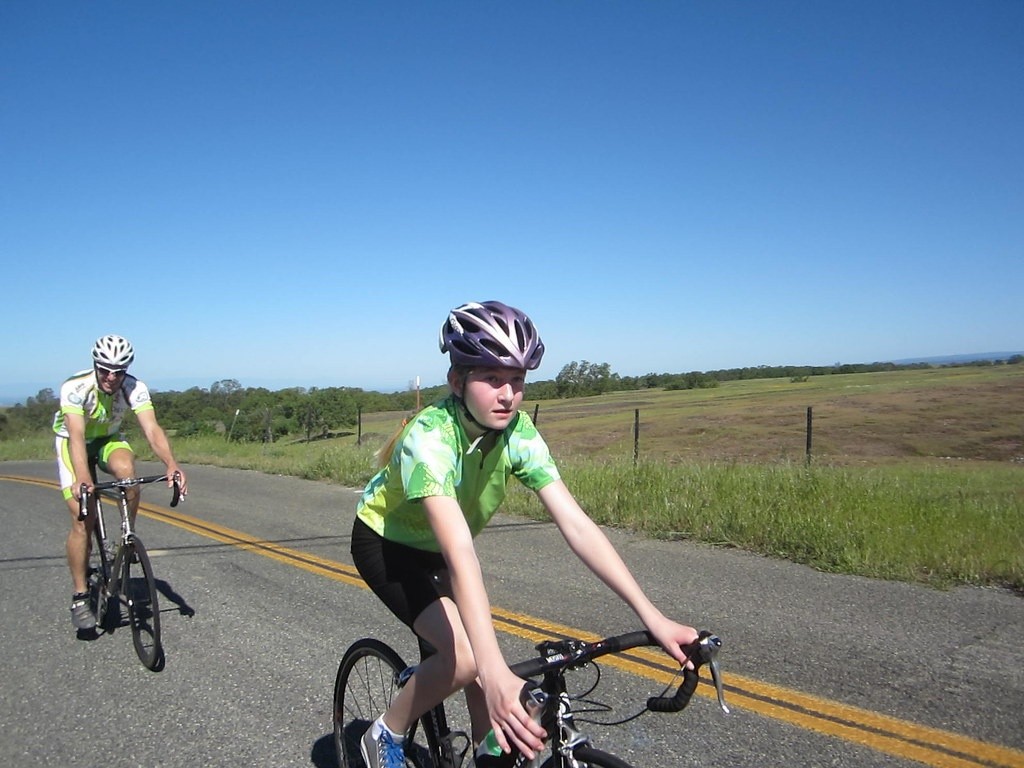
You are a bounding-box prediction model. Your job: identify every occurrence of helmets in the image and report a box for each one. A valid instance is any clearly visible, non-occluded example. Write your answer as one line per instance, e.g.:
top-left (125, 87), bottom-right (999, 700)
top-left (91, 335), bottom-right (135, 372)
top-left (439, 301), bottom-right (545, 370)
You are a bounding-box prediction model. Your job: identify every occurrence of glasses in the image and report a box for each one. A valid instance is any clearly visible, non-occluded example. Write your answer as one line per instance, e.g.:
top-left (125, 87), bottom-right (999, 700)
top-left (96, 367), bottom-right (125, 376)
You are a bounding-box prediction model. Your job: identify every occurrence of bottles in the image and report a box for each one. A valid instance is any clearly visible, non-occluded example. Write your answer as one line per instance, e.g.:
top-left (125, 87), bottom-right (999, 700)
top-left (466, 728), bottom-right (502, 768)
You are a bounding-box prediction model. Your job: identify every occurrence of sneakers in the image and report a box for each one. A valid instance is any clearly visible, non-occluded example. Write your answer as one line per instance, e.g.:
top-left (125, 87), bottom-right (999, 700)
top-left (120, 541), bottom-right (141, 563)
top-left (71, 592), bottom-right (96, 629)
top-left (361, 713), bottom-right (407, 768)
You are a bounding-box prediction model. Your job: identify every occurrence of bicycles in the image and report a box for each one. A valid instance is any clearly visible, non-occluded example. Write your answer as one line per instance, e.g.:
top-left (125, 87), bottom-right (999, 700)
top-left (332, 624), bottom-right (730, 768)
top-left (72, 470), bottom-right (183, 670)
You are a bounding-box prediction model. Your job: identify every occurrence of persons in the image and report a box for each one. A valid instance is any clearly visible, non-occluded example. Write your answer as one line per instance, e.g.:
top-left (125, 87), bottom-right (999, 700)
top-left (349, 301), bottom-right (698, 768)
top-left (52, 334), bottom-right (188, 629)
